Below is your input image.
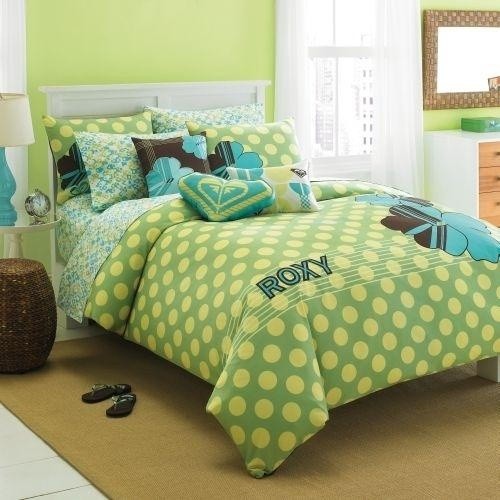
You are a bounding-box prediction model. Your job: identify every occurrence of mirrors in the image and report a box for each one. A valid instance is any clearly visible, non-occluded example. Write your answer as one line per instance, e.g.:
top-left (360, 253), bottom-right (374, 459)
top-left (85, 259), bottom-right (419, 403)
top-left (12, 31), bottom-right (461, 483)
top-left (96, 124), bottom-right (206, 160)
top-left (423, 11), bottom-right (500, 110)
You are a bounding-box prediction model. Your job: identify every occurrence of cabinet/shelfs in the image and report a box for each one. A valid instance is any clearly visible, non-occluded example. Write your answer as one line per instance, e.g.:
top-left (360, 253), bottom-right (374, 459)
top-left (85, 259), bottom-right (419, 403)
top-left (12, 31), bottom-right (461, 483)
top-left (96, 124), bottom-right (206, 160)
top-left (425, 130), bottom-right (500, 228)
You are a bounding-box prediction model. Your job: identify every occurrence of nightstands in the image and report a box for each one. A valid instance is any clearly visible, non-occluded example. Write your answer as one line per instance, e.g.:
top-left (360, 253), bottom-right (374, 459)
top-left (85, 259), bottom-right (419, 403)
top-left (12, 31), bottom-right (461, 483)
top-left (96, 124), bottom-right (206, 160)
top-left (0, 210), bottom-right (61, 258)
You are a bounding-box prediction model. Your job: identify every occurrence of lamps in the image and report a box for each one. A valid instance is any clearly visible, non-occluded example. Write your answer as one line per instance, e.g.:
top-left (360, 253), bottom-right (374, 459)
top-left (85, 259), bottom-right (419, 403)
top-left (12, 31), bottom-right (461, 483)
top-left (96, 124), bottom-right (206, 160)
top-left (0, 93), bottom-right (36, 226)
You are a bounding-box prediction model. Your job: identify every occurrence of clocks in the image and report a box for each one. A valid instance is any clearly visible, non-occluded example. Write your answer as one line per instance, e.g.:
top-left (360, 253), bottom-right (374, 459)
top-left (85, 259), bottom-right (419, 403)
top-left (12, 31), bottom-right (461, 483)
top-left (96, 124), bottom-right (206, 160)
top-left (25, 189), bottom-right (51, 225)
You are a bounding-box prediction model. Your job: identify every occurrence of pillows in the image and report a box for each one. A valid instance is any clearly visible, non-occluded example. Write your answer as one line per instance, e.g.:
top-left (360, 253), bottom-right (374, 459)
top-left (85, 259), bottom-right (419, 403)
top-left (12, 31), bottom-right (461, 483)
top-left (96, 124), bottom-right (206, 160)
top-left (42, 104), bottom-right (323, 222)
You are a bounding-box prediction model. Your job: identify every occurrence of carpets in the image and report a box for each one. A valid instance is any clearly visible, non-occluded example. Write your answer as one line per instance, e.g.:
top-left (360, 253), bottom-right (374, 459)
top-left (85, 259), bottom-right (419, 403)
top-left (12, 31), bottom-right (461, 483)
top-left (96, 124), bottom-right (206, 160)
top-left (0, 334), bottom-right (500, 500)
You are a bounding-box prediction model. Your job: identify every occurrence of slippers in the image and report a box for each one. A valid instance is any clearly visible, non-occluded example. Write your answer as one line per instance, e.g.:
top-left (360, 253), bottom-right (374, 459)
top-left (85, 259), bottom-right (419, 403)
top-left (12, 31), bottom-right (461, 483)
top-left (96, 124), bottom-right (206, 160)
top-left (108, 392), bottom-right (135, 418)
top-left (81, 383), bottom-right (131, 403)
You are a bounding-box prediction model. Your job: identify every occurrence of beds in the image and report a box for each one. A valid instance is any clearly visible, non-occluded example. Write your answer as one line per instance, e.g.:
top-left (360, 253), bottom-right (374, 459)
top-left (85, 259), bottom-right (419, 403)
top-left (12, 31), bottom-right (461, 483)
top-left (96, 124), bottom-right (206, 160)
top-left (39, 80), bottom-right (500, 479)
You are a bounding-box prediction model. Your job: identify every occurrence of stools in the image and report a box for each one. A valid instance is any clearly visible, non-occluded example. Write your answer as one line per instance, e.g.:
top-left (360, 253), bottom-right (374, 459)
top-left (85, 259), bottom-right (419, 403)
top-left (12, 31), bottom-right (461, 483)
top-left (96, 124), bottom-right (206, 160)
top-left (0, 258), bottom-right (57, 374)
